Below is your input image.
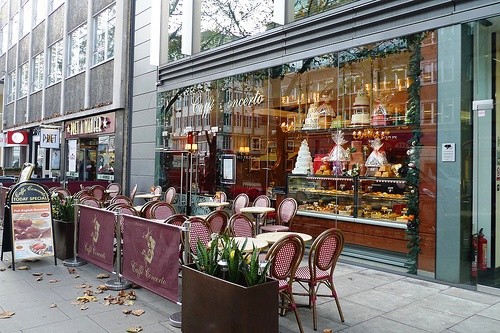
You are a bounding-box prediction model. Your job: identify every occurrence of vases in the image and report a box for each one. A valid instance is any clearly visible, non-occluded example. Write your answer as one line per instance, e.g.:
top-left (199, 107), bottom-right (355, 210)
top-left (181, 263), bottom-right (279, 333)
top-left (52, 218), bottom-right (80, 261)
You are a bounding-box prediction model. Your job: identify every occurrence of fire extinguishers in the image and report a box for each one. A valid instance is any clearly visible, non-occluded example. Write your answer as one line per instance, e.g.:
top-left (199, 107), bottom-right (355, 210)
top-left (472, 233), bottom-right (478, 277)
top-left (477, 228), bottom-right (488, 270)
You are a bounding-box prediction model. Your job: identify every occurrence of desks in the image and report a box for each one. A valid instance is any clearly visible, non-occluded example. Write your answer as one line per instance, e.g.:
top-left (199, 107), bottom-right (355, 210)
top-left (104, 190), bottom-right (118, 202)
top-left (239, 207), bottom-right (275, 235)
top-left (208, 237), bottom-right (269, 272)
top-left (198, 202), bottom-right (230, 213)
top-left (255, 232), bottom-right (313, 317)
top-left (134, 194), bottom-right (162, 202)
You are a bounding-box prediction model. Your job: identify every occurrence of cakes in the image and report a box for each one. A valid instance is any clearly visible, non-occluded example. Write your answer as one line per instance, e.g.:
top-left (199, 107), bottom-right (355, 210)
top-left (353, 88), bottom-right (370, 105)
top-left (350, 113), bottom-right (370, 125)
top-left (303, 103), bottom-right (320, 128)
top-left (371, 113), bottom-right (394, 126)
top-left (292, 140), bottom-right (314, 175)
top-left (347, 140), bottom-right (365, 176)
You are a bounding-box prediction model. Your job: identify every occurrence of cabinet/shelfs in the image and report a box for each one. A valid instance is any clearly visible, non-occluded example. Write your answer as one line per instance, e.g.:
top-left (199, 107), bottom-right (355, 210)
top-left (285, 173), bottom-right (409, 224)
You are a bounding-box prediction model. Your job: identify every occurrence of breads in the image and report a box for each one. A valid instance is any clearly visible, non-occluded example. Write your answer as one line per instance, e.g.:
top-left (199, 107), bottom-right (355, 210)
top-left (315, 165), bottom-right (331, 175)
top-left (298, 186), bottom-right (409, 222)
top-left (374, 166), bottom-right (394, 178)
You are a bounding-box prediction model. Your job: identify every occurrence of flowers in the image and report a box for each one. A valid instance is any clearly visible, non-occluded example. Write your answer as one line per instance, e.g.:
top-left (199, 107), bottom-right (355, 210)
top-left (191, 226), bottom-right (272, 288)
top-left (49, 190), bottom-right (82, 223)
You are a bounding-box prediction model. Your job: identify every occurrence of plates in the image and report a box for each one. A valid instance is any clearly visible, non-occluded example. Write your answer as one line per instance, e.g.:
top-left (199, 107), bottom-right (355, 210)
top-left (29, 242), bottom-right (52, 256)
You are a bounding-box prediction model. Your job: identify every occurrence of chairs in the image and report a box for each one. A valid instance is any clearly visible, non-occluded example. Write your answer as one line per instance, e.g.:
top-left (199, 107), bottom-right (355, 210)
top-left (48, 182), bottom-right (345, 333)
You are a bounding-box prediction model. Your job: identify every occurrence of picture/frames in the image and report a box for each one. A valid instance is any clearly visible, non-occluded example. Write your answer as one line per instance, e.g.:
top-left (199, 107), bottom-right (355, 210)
top-left (250, 157), bottom-right (260, 170)
top-left (285, 139), bottom-right (294, 153)
top-left (285, 159), bottom-right (294, 171)
top-left (251, 136), bottom-right (261, 150)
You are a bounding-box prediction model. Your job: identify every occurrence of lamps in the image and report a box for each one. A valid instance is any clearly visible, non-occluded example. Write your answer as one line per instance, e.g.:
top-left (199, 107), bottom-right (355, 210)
top-left (351, 59), bottom-right (391, 141)
top-left (185, 143), bottom-right (198, 161)
top-left (280, 73), bottom-right (308, 133)
top-left (237, 146), bottom-right (250, 162)
